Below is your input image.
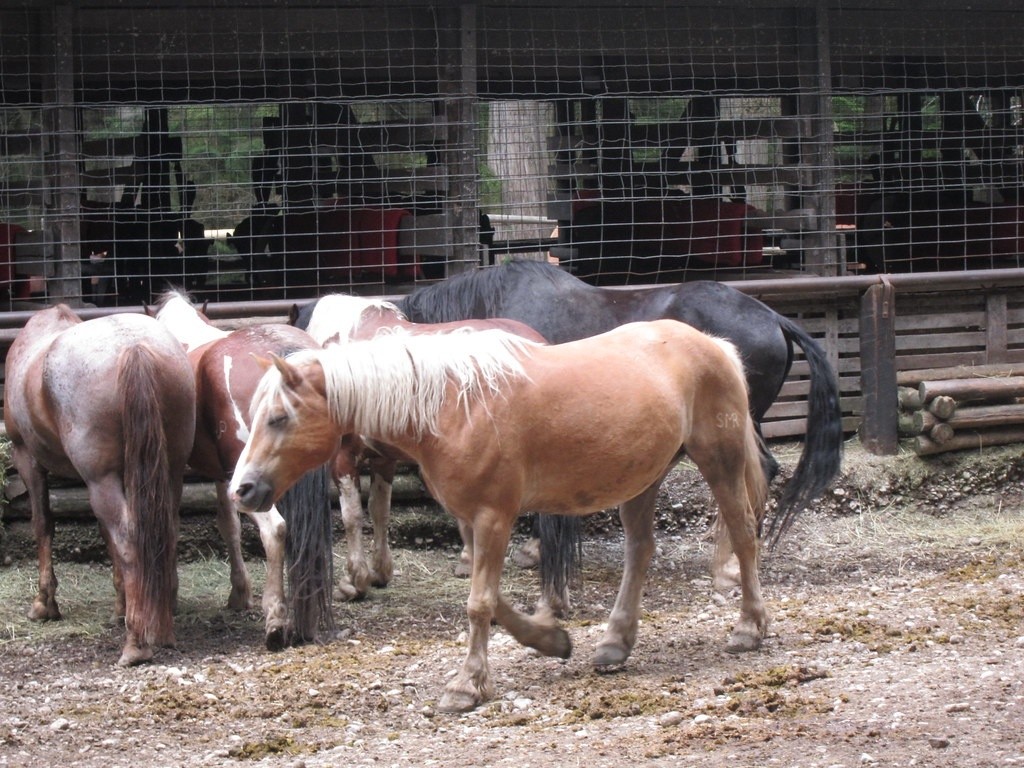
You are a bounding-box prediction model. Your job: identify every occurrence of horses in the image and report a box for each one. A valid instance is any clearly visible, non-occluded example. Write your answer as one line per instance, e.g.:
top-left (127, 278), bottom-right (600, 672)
top-left (2, 302), bottom-right (198, 669)
top-left (304, 292), bottom-right (584, 623)
top-left (225, 317), bottom-right (769, 716)
top-left (141, 279), bottom-right (336, 653)
top-left (286, 259), bottom-right (844, 627)
